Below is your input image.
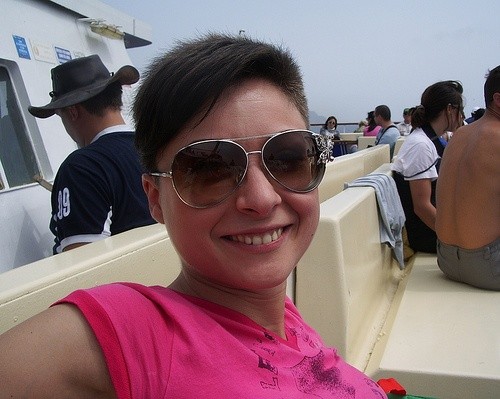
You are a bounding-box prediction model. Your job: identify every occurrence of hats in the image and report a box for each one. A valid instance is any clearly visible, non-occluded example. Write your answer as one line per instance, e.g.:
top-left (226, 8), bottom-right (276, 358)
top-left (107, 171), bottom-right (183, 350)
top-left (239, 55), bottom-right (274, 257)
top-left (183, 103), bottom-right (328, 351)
top-left (27, 54), bottom-right (140, 118)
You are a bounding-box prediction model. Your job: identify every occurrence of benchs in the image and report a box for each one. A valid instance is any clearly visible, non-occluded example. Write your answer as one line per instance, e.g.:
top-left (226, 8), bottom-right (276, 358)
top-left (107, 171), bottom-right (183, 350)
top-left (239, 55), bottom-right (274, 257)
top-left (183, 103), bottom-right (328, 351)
top-left (294, 163), bottom-right (499, 398)
top-left (0, 132), bottom-right (407, 337)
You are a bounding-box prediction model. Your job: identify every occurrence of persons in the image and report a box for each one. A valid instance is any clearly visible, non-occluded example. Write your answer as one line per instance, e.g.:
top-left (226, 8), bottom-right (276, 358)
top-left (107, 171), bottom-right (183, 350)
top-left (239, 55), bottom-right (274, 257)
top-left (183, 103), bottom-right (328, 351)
top-left (0, 34), bottom-right (388, 399)
top-left (435, 66), bottom-right (500, 291)
top-left (28, 55), bottom-right (158, 255)
top-left (320, 80), bottom-right (486, 254)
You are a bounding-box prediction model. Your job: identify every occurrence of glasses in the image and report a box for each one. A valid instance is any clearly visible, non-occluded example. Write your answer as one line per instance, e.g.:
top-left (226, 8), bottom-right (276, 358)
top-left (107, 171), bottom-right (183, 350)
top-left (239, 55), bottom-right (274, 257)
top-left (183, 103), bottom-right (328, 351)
top-left (146, 129), bottom-right (334, 210)
top-left (328, 121), bottom-right (336, 125)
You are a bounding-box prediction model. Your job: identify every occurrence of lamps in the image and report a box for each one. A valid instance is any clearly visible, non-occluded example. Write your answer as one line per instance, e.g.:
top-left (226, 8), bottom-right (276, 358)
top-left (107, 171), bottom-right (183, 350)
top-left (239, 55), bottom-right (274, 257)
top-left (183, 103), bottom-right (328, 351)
top-left (77, 18), bottom-right (126, 40)
